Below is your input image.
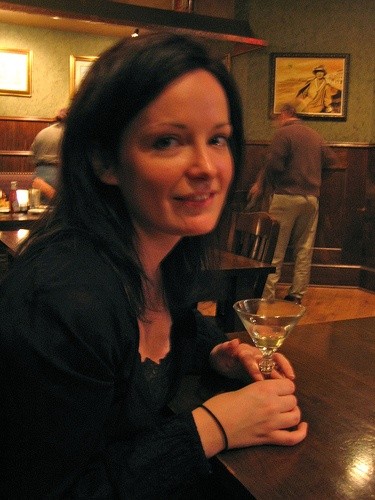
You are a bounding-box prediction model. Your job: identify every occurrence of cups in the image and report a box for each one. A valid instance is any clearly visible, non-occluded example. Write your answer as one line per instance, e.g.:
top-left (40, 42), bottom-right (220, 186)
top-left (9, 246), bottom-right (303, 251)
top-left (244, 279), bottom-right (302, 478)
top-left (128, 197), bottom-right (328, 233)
top-left (30, 189), bottom-right (41, 208)
top-left (16, 190), bottom-right (29, 211)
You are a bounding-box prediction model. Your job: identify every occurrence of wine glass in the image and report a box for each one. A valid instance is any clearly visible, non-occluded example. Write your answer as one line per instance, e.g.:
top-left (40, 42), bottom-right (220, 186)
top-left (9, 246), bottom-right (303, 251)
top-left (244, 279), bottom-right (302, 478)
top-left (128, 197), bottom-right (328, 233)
top-left (232, 298), bottom-right (305, 380)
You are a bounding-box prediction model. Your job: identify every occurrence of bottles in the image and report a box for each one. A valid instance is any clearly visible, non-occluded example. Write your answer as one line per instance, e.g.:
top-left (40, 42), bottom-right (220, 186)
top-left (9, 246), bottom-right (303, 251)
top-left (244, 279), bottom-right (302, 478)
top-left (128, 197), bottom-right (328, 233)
top-left (8, 181), bottom-right (19, 213)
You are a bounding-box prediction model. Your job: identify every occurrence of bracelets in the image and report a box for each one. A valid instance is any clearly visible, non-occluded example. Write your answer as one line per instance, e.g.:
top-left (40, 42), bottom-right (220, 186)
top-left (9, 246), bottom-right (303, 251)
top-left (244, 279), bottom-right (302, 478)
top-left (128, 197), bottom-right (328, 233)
top-left (198, 404), bottom-right (228, 452)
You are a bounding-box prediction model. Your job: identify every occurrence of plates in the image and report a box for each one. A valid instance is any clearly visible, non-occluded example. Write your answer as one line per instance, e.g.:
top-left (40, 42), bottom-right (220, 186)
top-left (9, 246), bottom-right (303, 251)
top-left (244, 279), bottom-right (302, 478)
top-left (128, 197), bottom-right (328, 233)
top-left (29, 209), bottom-right (46, 213)
top-left (0, 208), bottom-right (10, 213)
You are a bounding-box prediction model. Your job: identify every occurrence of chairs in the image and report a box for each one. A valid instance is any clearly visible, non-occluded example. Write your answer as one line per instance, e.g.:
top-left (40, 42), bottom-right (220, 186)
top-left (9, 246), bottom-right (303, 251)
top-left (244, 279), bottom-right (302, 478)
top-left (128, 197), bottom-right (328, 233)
top-left (202, 211), bottom-right (281, 333)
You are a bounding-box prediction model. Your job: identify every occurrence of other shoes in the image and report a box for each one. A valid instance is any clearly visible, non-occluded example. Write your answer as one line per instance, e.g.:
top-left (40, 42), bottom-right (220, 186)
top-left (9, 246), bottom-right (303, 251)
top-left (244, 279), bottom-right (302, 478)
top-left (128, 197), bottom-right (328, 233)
top-left (284, 295), bottom-right (302, 305)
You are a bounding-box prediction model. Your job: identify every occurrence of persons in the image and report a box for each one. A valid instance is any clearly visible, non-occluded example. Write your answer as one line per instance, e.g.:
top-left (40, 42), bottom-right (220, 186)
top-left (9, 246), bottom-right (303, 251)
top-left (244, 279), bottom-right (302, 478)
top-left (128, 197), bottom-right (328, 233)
top-left (296, 65), bottom-right (334, 113)
top-left (27, 108), bottom-right (70, 201)
top-left (0, 30), bottom-right (308, 500)
top-left (248, 102), bottom-right (337, 305)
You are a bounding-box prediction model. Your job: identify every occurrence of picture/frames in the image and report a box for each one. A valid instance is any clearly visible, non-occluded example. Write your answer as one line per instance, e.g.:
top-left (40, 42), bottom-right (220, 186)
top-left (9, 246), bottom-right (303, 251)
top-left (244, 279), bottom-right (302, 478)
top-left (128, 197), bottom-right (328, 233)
top-left (69, 54), bottom-right (99, 100)
top-left (267, 52), bottom-right (351, 122)
top-left (0, 48), bottom-right (33, 98)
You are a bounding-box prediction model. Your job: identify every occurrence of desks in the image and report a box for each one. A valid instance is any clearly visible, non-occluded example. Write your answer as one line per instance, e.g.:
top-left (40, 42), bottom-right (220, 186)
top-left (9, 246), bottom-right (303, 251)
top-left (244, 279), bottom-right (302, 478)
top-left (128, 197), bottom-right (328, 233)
top-left (200, 246), bottom-right (276, 300)
top-left (0, 195), bottom-right (57, 258)
top-left (167, 317), bottom-right (375, 500)
